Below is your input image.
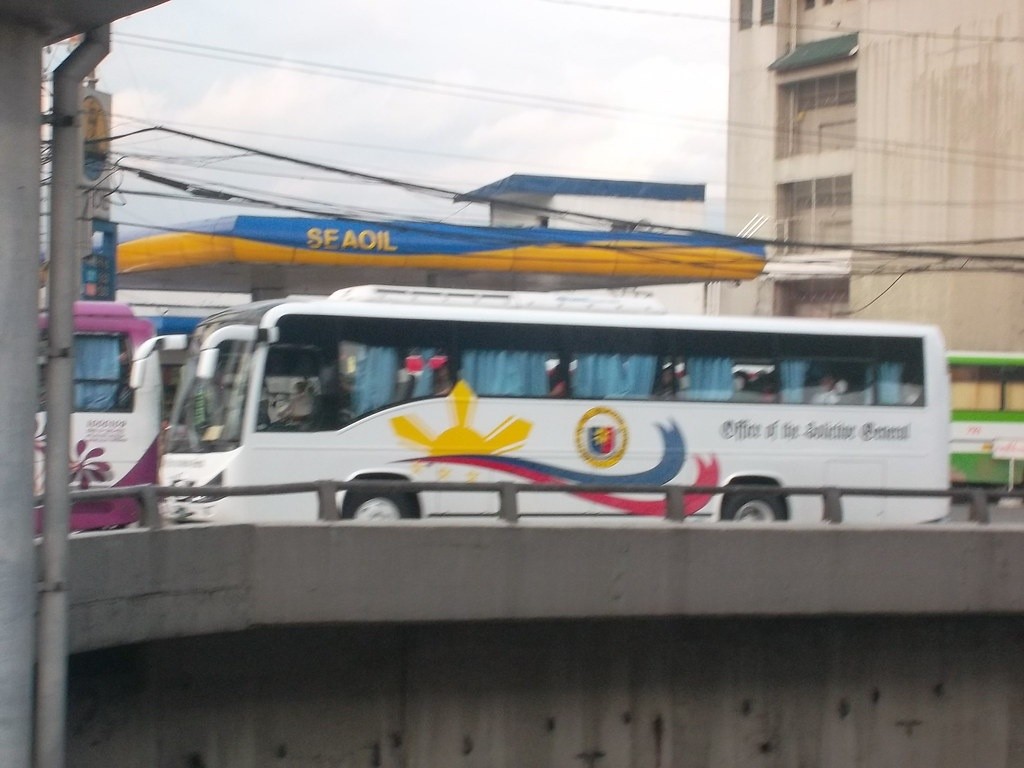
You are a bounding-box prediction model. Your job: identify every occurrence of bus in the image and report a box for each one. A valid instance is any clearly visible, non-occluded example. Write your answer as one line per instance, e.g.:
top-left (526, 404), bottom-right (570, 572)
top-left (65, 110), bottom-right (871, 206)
top-left (944, 349), bottom-right (1024, 504)
top-left (33, 303), bottom-right (164, 535)
top-left (128, 284), bottom-right (953, 523)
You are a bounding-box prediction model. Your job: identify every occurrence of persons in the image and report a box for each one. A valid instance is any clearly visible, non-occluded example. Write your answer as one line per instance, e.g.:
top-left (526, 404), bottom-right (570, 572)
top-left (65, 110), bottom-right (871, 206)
top-left (657, 367), bottom-right (677, 395)
top-left (811, 373), bottom-right (841, 404)
top-left (549, 365), bottom-right (570, 398)
top-left (733, 370), bottom-right (779, 403)
top-left (277, 382), bottom-right (318, 419)
top-left (434, 364), bottom-right (454, 398)
